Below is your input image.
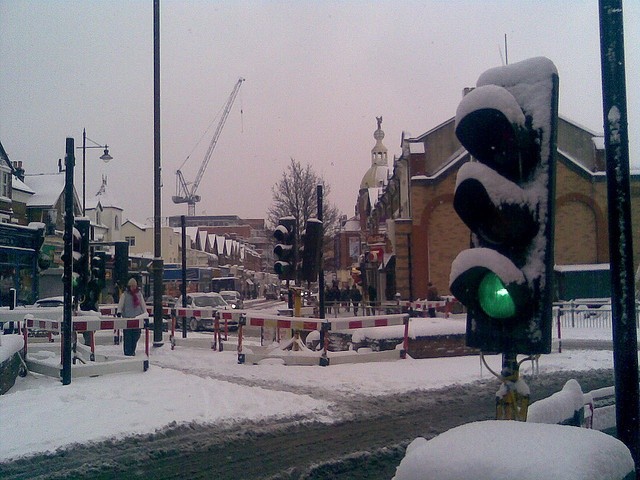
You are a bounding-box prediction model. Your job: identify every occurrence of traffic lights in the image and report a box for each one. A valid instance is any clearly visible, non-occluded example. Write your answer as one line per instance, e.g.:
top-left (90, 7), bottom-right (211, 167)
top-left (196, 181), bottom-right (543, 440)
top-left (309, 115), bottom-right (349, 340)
top-left (447, 56), bottom-right (561, 356)
top-left (60, 220), bottom-right (92, 294)
top-left (92, 251), bottom-right (104, 282)
top-left (273, 219), bottom-right (299, 280)
top-left (298, 217), bottom-right (324, 283)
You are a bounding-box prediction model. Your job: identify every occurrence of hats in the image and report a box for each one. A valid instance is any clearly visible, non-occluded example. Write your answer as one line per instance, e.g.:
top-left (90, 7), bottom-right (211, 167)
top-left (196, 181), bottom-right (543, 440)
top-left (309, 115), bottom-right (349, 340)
top-left (128, 278), bottom-right (137, 286)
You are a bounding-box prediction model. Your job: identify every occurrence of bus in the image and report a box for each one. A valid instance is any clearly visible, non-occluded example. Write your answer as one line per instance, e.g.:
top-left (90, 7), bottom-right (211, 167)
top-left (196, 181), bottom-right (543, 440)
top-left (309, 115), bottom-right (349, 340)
top-left (211, 277), bottom-right (242, 297)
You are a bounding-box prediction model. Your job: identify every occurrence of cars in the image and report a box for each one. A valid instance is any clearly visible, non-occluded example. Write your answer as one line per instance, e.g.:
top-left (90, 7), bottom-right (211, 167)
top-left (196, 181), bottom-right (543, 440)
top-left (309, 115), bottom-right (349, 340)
top-left (34, 297), bottom-right (76, 307)
top-left (145, 294), bottom-right (176, 320)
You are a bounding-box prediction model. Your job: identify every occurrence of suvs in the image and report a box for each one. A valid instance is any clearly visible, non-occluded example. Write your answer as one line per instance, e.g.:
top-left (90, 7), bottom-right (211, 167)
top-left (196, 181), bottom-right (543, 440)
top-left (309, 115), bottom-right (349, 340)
top-left (220, 290), bottom-right (243, 309)
top-left (172, 291), bottom-right (238, 332)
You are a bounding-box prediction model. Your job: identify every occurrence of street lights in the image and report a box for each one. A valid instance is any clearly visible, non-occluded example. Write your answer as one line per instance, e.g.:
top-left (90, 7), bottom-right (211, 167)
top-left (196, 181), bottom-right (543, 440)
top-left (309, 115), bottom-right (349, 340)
top-left (76, 127), bottom-right (113, 217)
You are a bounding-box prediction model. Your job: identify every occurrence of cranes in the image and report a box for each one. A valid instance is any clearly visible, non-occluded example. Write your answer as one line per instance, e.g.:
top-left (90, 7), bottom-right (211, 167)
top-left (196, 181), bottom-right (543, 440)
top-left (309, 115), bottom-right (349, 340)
top-left (172, 76), bottom-right (246, 217)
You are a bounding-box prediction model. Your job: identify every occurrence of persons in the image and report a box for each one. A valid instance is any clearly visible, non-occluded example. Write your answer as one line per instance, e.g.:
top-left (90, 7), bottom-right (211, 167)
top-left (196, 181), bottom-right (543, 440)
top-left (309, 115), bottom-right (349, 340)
top-left (325, 282), bottom-right (378, 316)
top-left (117, 278), bottom-right (147, 360)
